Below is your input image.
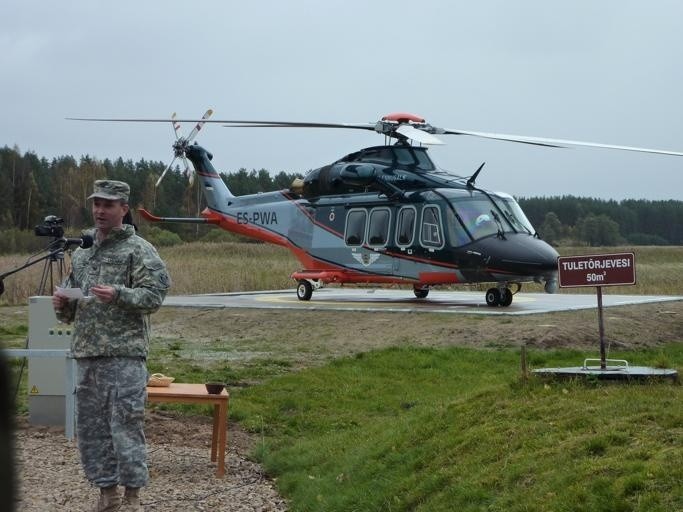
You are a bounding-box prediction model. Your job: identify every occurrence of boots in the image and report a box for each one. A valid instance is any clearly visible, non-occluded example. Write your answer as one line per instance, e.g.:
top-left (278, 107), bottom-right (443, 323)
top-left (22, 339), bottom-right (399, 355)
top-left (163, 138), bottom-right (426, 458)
top-left (83, 484), bottom-right (122, 512)
top-left (117, 487), bottom-right (141, 512)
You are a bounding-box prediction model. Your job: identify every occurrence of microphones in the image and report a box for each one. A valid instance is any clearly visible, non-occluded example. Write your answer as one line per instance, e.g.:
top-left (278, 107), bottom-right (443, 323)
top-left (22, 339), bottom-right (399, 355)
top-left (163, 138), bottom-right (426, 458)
top-left (66, 235), bottom-right (93, 249)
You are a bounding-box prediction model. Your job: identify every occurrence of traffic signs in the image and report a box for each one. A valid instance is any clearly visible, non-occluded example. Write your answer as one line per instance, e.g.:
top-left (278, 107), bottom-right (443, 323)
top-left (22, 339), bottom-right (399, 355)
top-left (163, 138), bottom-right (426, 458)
top-left (556, 251), bottom-right (638, 289)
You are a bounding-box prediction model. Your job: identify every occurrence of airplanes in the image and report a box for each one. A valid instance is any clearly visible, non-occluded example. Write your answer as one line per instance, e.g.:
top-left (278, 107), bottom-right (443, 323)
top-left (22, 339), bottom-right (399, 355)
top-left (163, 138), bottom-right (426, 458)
top-left (64, 109), bottom-right (682, 307)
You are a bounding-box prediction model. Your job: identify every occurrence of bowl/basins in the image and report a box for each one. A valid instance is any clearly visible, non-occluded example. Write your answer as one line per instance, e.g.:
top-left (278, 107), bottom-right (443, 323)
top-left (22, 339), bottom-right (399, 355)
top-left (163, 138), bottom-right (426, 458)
top-left (205, 382), bottom-right (227, 394)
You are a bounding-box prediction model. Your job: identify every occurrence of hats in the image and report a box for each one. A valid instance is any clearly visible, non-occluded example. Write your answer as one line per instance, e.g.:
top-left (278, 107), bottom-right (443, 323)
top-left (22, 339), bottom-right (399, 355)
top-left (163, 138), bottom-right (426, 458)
top-left (87, 178), bottom-right (131, 204)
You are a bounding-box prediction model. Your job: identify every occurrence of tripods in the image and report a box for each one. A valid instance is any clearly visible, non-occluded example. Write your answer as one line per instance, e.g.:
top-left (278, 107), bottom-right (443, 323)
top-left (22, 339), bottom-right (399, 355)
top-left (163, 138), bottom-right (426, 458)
top-left (10, 254), bottom-right (68, 415)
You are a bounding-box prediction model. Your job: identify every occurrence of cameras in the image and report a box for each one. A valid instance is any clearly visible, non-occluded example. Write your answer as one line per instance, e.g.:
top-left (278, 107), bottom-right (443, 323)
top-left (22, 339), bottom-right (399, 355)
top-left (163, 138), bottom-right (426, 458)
top-left (34, 214), bottom-right (63, 238)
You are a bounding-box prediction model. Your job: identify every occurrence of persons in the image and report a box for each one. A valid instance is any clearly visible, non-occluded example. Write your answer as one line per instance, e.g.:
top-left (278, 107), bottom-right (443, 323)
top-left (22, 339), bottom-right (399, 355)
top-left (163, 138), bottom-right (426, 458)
top-left (52, 179), bottom-right (172, 511)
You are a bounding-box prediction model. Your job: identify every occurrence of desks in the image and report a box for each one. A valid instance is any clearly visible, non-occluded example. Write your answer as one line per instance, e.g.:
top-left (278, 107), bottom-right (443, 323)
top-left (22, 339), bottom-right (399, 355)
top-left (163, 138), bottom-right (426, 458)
top-left (143, 383), bottom-right (229, 479)
